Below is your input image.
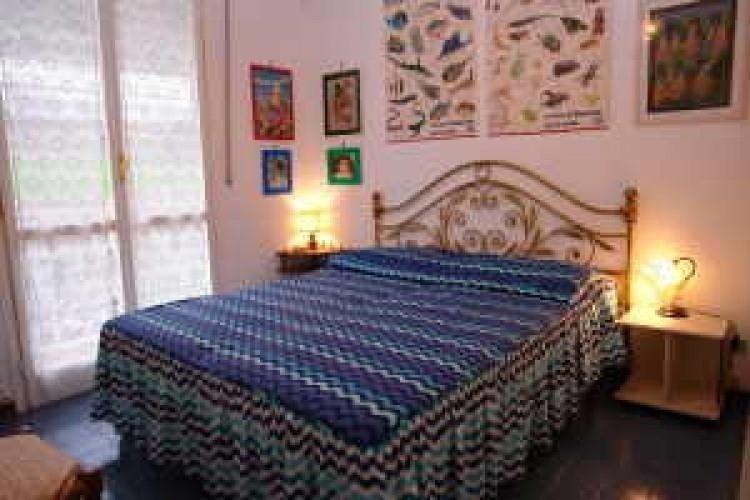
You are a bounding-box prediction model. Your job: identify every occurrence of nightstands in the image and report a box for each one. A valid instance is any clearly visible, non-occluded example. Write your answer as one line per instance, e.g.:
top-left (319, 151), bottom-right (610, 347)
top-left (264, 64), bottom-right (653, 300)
top-left (275, 248), bottom-right (338, 277)
top-left (613, 306), bottom-right (736, 422)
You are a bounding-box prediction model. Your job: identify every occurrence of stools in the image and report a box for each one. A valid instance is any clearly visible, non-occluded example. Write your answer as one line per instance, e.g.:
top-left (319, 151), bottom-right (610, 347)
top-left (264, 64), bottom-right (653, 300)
top-left (0, 399), bottom-right (103, 500)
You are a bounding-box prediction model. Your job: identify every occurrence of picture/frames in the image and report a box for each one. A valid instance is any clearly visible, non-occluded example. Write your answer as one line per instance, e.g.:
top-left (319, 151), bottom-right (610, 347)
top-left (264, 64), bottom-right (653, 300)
top-left (250, 65), bottom-right (294, 140)
top-left (261, 150), bottom-right (292, 195)
top-left (322, 69), bottom-right (364, 137)
top-left (639, 0), bottom-right (742, 122)
top-left (326, 147), bottom-right (361, 185)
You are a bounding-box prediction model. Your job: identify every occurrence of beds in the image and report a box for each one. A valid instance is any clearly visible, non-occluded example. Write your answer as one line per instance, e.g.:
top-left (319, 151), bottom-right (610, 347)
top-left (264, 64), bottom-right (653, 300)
top-left (91, 161), bottom-right (639, 500)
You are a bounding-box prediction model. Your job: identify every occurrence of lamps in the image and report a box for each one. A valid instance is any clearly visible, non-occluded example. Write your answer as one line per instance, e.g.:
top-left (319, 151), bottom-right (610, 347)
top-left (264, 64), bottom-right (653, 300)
top-left (286, 188), bottom-right (336, 249)
top-left (644, 256), bottom-right (696, 318)
